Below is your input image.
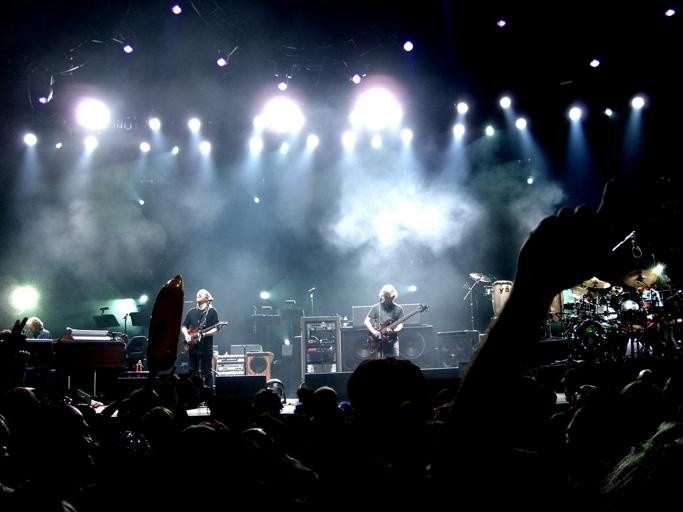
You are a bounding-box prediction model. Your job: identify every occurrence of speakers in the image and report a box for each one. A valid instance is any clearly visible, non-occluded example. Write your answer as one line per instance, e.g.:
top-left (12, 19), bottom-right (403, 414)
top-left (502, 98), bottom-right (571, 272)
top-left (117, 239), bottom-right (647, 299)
top-left (341, 324), bottom-right (379, 372)
top-left (398, 324), bottom-right (434, 368)
top-left (215, 376), bottom-right (265, 407)
top-left (305, 372), bottom-right (352, 403)
top-left (245, 352), bottom-right (272, 382)
top-left (436, 331), bottom-right (479, 367)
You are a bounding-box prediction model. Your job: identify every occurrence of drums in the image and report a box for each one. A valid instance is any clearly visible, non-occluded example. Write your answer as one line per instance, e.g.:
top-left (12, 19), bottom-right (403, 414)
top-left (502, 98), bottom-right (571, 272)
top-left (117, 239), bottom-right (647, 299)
top-left (619, 291), bottom-right (641, 312)
top-left (596, 304), bottom-right (618, 322)
top-left (482, 285), bottom-right (493, 296)
top-left (623, 308), bottom-right (648, 330)
top-left (491, 280), bottom-right (513, 316)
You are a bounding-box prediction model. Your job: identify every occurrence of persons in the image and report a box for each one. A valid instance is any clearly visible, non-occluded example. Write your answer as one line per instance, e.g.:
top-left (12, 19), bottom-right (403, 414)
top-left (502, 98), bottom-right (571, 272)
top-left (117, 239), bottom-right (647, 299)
top-left (2, 314), bottom-right (682, 512)
top-left (363, 283), bottom-right (405, 363)
top-left (443, 176), bottom-right (625, 421)
top-left (181, 289), bottom-right (219, 389)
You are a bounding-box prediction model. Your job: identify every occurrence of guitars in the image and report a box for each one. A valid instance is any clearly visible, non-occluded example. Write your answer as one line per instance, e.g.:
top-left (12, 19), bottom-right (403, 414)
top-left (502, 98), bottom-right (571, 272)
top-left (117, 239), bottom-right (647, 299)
top-left (367, 303), bottom-right (431, 347)
top-left (181, 321), bottom-right (230, 352)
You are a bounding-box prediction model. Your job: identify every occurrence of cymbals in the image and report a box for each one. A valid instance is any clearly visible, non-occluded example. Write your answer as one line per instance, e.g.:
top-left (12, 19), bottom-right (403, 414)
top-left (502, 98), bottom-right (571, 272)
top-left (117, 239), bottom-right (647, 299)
top-left (623, 268), bottom-right (657, 287)
top-left (583, 279), bottom-right (610, 290)
top-left (469, 272), bottom-right (492, 284)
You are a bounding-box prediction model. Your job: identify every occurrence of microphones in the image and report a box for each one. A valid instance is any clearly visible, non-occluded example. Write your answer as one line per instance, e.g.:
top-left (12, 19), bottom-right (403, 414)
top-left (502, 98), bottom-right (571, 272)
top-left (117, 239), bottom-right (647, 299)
top-left (285, 300), bottom-right (295, 304)
top-left (205, 299), bottom-right (213, 302)
top-left (100, 307), bottom-right (109, 310)
top-left (380, 297), bottom-right (385, 303)
top-left (308, 288), bottom-right (316, 293)
top-left (123, 313), bottom-right (128, 319)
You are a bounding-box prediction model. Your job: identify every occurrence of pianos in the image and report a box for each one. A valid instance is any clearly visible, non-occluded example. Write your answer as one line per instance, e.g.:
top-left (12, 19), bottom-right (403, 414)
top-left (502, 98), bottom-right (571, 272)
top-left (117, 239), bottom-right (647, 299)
top-left (24, 336), bottom-right (123, 380)
